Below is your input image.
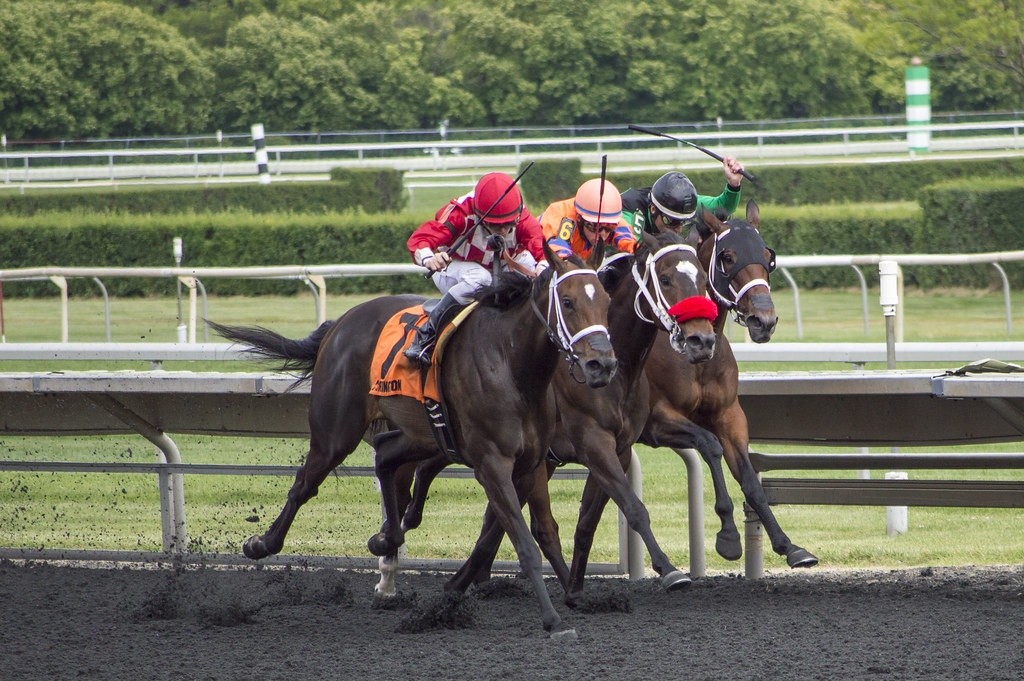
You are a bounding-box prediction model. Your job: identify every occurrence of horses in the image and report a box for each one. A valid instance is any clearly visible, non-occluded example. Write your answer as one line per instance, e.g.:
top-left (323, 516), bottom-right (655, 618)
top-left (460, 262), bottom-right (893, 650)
top-left (198, 196), bottom-right (820, 644)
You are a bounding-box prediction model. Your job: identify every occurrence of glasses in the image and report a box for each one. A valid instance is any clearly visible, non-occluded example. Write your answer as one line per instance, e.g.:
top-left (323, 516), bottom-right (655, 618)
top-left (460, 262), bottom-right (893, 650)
top-left (657, 210), bottom-right (684, 227)
top-left (482, 221), bottom-right (517, 230)
top-left (581, 218), bottom-right (617, 234)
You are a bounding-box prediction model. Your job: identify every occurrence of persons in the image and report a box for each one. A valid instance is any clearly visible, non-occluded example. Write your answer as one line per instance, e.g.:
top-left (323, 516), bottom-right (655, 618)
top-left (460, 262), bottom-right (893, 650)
top-left (403, 172), bottom-right (550, 366)
top-left (620, 155), bottom-right (745, 253)
top-left (531, 177), bottom-right (642, 278)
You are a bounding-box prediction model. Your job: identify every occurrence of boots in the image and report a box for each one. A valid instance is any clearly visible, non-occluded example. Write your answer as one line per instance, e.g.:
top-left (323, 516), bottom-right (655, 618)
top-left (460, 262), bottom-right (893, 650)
top-left (402, 292), bottom-right (464, 368)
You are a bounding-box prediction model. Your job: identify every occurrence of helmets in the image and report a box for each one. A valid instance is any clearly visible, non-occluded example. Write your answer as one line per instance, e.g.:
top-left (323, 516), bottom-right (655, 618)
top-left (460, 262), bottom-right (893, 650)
top-left (648, 170), bottom-right (697, 219)
top-left (474, 174), bottom-right (523, 223)
top-left (575, 178), bottom-right (622, 223)
